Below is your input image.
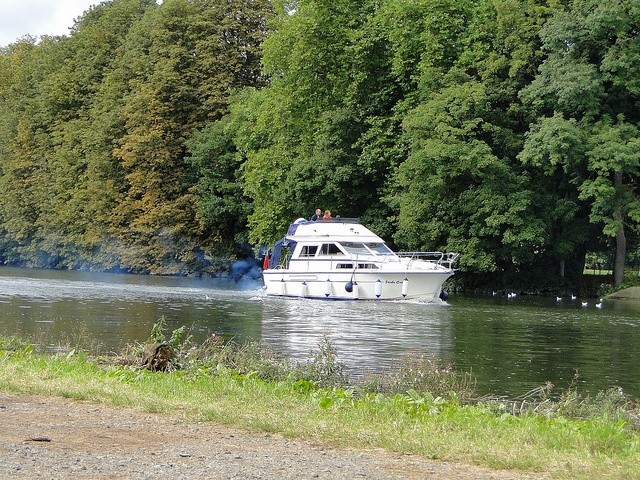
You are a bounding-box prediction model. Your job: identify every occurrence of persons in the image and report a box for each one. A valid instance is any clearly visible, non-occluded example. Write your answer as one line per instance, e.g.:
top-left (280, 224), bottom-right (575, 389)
top-left (323, 210), bottom-right (333, 220)
top-left (311, 209), bottom-right (321, 219)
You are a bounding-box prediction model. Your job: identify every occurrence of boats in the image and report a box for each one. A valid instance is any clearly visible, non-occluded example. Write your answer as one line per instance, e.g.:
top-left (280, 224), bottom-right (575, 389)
top-left (263, 218), bottom-right (458, 302)
